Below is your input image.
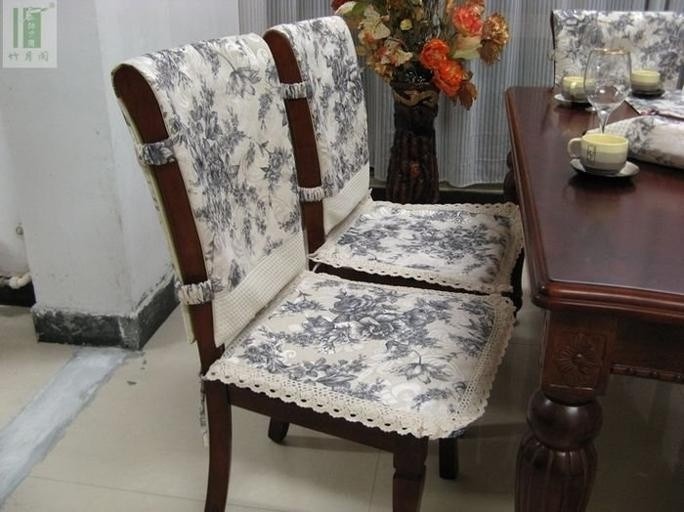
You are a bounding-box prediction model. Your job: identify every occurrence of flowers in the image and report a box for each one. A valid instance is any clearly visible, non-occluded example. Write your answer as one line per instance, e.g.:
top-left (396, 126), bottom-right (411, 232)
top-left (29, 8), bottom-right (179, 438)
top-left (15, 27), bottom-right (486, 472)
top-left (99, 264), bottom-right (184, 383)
top-left (332, 0), bottom-right (507, 112)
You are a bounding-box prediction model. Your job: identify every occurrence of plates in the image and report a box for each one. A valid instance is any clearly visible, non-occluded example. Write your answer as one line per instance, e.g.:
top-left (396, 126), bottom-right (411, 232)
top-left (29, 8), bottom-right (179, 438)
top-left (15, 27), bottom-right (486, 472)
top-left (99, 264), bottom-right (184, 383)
top-left (632, 88), bottom-right (666, 98)
top-left (553, 93), bottom-right (589, 106)
top-left (569, 158), bottom-right (640, 180)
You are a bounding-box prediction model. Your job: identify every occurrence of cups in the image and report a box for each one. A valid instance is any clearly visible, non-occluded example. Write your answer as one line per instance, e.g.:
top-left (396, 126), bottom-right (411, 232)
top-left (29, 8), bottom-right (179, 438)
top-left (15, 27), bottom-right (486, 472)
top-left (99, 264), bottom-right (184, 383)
top-left (567, 133), bottom-right (630, 176)
top-left (629, 69), bottom-right (661, 93)
top-left (561, 76), bottom-right (593, 100)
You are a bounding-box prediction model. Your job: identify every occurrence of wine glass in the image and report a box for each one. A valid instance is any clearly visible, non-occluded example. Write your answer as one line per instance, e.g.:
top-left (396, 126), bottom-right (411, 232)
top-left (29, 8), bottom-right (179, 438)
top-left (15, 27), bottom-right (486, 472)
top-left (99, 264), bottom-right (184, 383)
top-left (581, 49), bottom-right (632, 133)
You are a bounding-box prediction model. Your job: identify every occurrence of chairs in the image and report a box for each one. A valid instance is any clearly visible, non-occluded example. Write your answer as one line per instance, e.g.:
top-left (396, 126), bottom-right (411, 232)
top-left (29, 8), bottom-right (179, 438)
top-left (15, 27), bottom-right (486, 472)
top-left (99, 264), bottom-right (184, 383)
top-left (549, 7), bottom-right (683, 93)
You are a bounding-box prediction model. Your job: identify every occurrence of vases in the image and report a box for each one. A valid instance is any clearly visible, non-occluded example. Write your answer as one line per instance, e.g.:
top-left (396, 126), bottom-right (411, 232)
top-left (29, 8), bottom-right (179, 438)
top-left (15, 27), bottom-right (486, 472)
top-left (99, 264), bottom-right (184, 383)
top-left (385, 77), bottom-right (442, 204)
